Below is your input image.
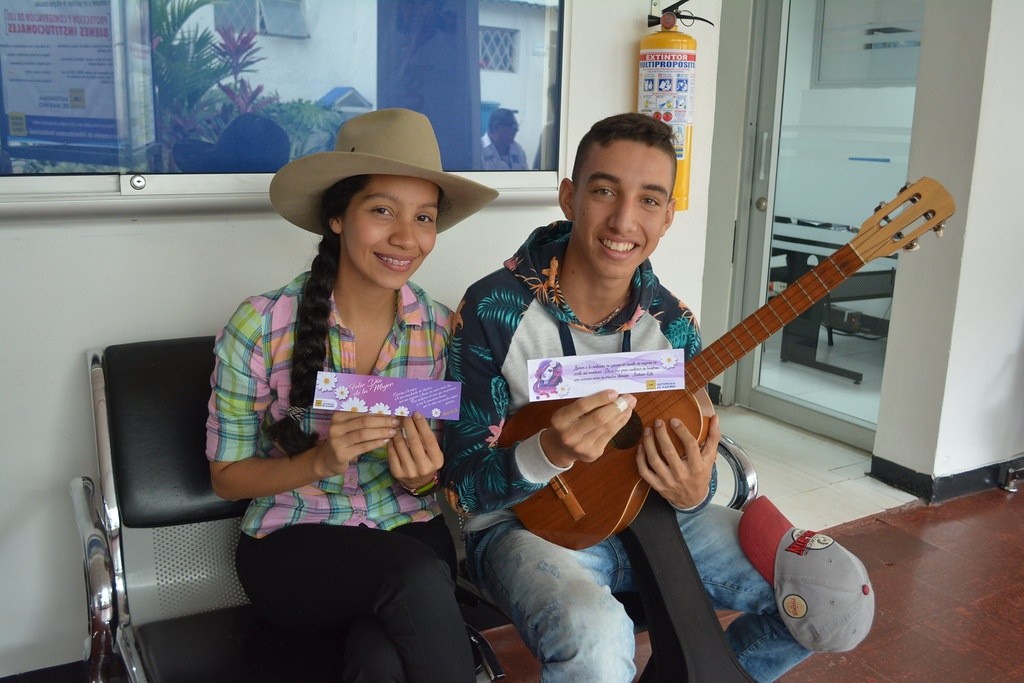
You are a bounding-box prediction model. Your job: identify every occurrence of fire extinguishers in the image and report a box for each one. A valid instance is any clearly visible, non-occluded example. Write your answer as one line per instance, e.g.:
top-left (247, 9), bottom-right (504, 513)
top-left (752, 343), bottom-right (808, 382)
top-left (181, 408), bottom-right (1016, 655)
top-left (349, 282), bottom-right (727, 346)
top-left (635, 0), bottom-right (715, 212)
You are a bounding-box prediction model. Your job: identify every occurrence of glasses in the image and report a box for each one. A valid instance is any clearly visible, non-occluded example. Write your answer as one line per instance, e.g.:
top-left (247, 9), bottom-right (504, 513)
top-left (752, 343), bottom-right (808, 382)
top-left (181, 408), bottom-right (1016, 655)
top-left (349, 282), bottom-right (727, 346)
top-left (500, 122), bottom-right (519, 129)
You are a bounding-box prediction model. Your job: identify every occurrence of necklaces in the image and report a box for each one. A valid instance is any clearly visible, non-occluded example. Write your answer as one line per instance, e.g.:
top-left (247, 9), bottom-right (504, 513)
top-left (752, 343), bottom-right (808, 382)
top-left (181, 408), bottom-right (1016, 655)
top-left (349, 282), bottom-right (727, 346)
top-left (591, 291), bottom-right (631, 325)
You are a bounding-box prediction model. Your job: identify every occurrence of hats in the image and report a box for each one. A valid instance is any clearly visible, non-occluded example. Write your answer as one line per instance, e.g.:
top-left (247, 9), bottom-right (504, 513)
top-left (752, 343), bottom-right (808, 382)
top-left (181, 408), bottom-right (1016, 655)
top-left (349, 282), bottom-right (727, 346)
top-left (269, 108), bottom-right (500, 236)
top-left (173, 112), bottom-right (291, 173)
top-left (739, 494), bottom-right (875, 654)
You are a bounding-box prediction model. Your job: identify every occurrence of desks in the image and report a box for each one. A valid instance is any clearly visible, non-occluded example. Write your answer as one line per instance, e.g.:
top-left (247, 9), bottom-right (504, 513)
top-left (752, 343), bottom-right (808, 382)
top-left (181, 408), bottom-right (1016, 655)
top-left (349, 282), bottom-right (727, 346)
top-left (773, 217), bottom-right (901, 384)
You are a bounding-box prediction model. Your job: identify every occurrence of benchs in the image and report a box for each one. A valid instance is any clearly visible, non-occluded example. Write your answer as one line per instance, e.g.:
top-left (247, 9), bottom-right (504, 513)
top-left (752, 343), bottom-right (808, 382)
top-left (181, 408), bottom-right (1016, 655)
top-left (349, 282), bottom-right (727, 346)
top-left (72, 330), bottom-right (760, 683)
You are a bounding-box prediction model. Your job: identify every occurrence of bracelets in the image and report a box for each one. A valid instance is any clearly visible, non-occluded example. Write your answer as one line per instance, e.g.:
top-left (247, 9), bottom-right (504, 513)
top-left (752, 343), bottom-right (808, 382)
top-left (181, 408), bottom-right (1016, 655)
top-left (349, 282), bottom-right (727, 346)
top-left (401, 471), bottom-right (438, 495)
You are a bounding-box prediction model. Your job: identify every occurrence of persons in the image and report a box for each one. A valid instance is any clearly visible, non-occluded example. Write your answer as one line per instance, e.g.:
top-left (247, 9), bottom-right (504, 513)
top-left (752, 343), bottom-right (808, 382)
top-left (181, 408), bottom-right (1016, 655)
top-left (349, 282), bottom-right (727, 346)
top-left (438, 112), bottom-right (875, 683)
top-left (530, 82), bottom-right (556, 170)
top-left (205, 108), bottom-right (476, 683)
top-left (170, 109), bottom-right (290, 174)
top-left (480, 108), bottom-right (530, 170)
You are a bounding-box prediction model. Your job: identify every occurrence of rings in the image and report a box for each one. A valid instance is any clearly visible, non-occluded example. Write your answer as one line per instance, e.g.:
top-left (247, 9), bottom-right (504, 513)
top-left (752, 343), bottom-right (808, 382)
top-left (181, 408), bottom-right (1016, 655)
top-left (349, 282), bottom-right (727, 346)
top-left (614, 398), bottom-right (628, 412)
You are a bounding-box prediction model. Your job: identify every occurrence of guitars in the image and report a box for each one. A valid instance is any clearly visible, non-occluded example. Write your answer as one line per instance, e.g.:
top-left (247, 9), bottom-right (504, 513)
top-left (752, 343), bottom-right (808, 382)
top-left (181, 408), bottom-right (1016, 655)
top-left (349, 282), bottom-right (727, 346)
top-left (497, 173), bottom-right (957, 553)
